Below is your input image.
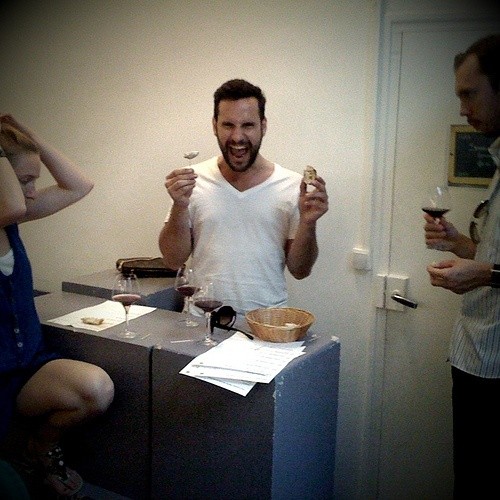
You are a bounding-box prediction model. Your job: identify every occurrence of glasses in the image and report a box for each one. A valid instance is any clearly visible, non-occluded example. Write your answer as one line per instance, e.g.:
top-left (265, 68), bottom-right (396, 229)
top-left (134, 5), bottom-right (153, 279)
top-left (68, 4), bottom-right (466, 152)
top-left (208, 306), bottom-right (253, 340)
top-left (469, 198), bottom-right (489, 244)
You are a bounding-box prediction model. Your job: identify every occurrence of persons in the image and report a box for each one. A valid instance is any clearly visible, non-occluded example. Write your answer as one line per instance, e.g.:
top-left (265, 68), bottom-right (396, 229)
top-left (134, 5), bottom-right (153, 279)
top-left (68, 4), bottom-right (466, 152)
top-left (0, 111), bottom-right (114, 498)
top-left (423, 33), bottom-right (500, 500)
top-left (158, 78), bottom-right (329, 324)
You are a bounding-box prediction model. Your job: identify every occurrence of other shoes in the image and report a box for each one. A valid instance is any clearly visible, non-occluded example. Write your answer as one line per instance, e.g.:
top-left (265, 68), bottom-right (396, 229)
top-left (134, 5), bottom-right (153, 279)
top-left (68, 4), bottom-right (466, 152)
top-left (46, 448), bottom-right (83, 494)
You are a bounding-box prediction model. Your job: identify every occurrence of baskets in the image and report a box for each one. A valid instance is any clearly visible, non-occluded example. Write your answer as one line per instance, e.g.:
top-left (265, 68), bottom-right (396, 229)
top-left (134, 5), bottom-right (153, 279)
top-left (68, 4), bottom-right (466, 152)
top-left (245, 307), bottom-right (315, 343)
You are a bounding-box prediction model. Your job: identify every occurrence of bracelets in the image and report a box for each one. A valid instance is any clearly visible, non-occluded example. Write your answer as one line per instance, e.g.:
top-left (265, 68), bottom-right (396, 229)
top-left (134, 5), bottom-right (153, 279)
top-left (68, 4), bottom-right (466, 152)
top-left (490, 264), bottom-right (500, 289)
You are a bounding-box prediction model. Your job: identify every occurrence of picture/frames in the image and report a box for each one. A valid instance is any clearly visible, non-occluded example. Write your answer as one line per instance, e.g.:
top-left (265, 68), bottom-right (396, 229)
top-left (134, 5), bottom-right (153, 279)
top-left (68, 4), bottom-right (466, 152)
top-left (447, 124), bottom-right (497, 189)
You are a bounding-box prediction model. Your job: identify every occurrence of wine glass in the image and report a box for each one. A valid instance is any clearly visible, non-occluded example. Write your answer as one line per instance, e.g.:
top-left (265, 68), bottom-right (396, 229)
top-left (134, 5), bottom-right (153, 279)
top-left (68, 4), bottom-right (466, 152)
top-left (174, 267), bottom-right (202, 328)
top-left (192, 282), bottom-right (223, 346)
top-left (111, 274), bottom-right (141, 339)
top-left (421, 185), bottom-right (452, 249)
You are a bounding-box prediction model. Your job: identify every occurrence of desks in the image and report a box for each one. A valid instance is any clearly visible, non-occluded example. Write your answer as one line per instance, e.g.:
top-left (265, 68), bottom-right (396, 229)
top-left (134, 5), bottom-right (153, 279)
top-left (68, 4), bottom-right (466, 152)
top-left (33, 268), bottom-right (341, 500)
